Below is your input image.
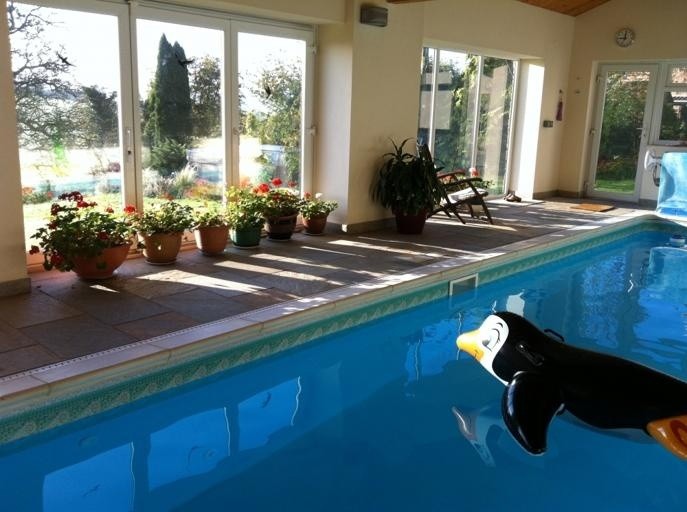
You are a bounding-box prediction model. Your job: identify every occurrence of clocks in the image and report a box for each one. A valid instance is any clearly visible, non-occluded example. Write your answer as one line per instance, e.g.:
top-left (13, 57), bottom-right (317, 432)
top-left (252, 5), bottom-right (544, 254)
top-left (615, 26), bottom-right (635, 47)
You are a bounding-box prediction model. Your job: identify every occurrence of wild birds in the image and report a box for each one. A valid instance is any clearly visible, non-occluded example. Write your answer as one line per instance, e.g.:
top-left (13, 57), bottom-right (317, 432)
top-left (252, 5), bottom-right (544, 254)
top-left (264, 85), bottom-right (271, 99)
top-left (173, 53), bottom-right (195, 69)
top-left (57, 52), bottom-right (71, 66)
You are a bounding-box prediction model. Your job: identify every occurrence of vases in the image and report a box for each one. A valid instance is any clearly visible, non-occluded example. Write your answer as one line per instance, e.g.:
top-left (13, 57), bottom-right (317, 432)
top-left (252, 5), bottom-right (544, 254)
top-left (265, 211), bottom-right (300, 241)
top-left (71, 240), bottom-right (133, 279)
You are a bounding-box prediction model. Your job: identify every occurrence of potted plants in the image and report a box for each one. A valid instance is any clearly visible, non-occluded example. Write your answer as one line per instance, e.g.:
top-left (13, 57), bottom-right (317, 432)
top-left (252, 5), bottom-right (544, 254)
top-left (135, 186), bottom-right (266, 265)
top-left (370, 136), bottom-right (446, 235)
top-left (301, 199), bottom-right (338, 236)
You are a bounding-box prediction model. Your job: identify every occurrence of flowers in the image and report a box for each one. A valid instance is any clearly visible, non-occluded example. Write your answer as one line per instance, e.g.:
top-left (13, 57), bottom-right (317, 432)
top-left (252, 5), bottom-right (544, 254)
top-left (29, 191), bottom-right (146, 270)
top-left (253, 178), bottom-right (311, 214)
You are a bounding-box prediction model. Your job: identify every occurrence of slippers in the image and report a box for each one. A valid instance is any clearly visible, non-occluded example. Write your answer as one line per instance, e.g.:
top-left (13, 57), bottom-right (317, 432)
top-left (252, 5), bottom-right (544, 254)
top-left (504, 193), bottom-right (521, 202)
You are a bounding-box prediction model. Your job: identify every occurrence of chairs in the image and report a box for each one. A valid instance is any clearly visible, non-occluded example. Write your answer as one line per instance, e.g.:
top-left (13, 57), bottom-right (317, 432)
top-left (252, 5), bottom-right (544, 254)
top-left (417, 140), bottom-right (495, 224)
